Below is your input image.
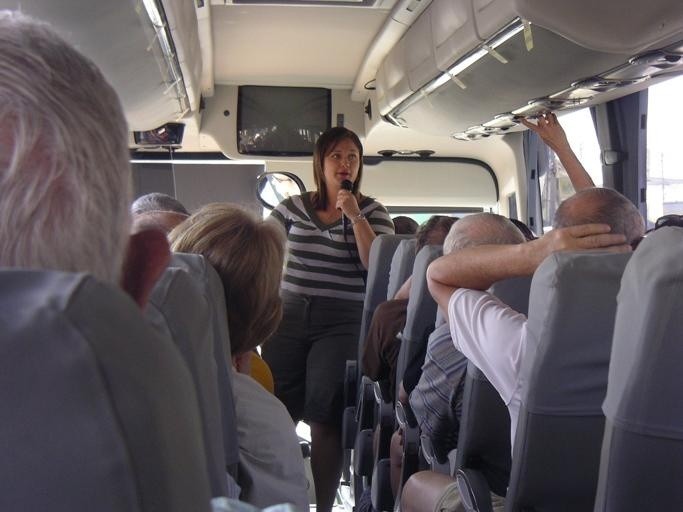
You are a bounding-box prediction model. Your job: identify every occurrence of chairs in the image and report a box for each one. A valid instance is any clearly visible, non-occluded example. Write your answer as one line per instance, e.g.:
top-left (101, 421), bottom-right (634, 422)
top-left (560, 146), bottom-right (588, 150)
top-left (593, 225), bottom-right (683, 512)
top-left (456, 249), bottom-right (637, 511)
top-left (0, 268), bottom-right (209, 512)
top-left (343, 234), bottom-right (516, 512)
top-left (145, 254), bottom-right (235, 512)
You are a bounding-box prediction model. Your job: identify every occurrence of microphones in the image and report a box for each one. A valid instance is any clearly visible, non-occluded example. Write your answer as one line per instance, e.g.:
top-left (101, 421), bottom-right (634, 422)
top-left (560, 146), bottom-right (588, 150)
top-left (341, 179), bottom-right (353, 238)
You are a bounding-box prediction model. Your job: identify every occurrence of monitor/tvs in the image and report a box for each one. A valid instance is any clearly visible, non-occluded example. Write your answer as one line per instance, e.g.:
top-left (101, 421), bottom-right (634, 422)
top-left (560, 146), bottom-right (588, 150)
top-left (237, 85), bottom-right (332, 156)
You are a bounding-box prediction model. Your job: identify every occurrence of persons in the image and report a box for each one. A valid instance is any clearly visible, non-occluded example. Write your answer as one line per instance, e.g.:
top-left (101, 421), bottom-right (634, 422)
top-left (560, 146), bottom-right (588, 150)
top-left (128, 193), bottom-right (309, 512)
top-left (262, 126), bottom-right (396, 512)
top-left (1, 9), bottom-right (172, 308)
top-left (359, 112), bottom-right (683, 512)
